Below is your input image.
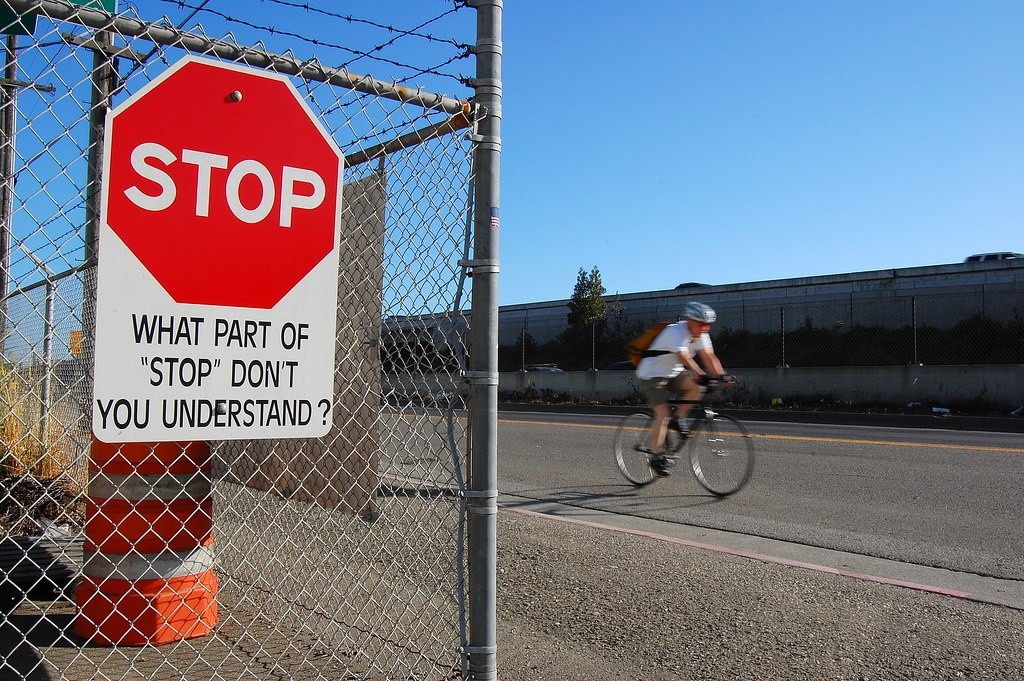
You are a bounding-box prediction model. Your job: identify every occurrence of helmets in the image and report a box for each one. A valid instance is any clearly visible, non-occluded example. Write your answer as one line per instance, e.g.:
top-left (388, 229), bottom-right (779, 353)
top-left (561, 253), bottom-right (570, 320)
top-left (683, 301), bottom-right (716, 323)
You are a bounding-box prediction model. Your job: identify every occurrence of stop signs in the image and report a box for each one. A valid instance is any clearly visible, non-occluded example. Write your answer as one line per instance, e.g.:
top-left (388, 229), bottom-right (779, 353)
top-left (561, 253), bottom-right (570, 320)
top-left (103, 50), bottom-right (339, 312)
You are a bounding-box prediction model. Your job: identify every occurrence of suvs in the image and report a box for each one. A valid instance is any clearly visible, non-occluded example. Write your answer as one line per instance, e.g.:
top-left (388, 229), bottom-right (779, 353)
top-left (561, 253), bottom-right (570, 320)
top-left (523, 364), bottom-right (565, 373)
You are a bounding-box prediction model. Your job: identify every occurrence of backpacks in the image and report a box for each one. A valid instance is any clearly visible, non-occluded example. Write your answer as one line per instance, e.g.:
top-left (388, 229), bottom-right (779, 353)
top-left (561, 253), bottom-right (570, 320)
top-left (628, 321), bottom-right (693, 366)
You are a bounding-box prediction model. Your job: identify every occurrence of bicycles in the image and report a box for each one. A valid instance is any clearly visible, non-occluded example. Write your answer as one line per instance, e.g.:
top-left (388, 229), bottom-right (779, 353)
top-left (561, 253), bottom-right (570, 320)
top-left (614, 376), bottom-right (755, 495)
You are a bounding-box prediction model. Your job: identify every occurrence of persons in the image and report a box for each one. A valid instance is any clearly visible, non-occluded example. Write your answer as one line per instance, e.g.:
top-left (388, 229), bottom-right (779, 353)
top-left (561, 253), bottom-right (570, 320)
top-left (635, 301), bottom-right (734, 479)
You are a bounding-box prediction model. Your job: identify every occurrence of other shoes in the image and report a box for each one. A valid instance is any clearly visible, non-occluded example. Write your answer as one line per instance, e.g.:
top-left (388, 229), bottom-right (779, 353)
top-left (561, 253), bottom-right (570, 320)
top-left (667, 414), bottom-right (690, 438)
top-left (646, 456), bottom-right (671, 477)
